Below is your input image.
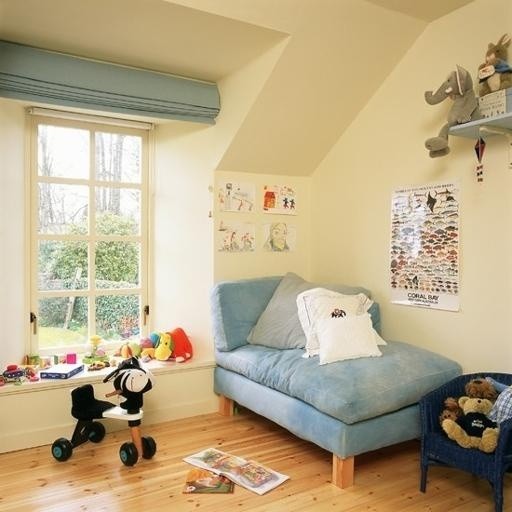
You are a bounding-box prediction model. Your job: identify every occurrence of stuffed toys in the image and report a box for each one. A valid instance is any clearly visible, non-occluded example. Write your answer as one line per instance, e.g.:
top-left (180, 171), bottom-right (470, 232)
top-left (475, 33), bottom-right (512, 97)
top-left (439, 378), bottom-right (499, 429)
top-left (442, 395), bottom-right (500, 454)
top-left (423, 62), bottom-right (479, 158)
top-left (113, 327), bottom-right (193, 364)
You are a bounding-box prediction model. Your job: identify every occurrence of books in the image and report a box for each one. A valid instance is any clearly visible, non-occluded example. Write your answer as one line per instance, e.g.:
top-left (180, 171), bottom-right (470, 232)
top-left (181, 467), bottom-right (234, 495)
top-left (182, 447), bottom-right (291, 497)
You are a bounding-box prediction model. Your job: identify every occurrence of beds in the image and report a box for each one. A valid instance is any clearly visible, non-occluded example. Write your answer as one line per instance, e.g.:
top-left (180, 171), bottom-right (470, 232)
top-left (210, 276), bottom-right (463, 490)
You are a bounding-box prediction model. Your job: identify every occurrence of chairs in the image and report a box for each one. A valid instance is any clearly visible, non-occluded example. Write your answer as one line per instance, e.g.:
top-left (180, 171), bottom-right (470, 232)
top-left (420, 373), bottom-right (512, 508)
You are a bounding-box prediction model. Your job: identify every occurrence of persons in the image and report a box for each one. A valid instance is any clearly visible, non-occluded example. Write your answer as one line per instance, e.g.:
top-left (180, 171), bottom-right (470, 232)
top-left (263, 222), bottom-right (290, 253)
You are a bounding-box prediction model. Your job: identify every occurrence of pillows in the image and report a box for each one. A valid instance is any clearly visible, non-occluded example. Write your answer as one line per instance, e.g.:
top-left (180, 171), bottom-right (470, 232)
top-left (317, 316), bottom-right (382, 365)
top-left (246, 271), bottom-right (373, 358)
top-left (295, 286), bottom-right (386, 357)
